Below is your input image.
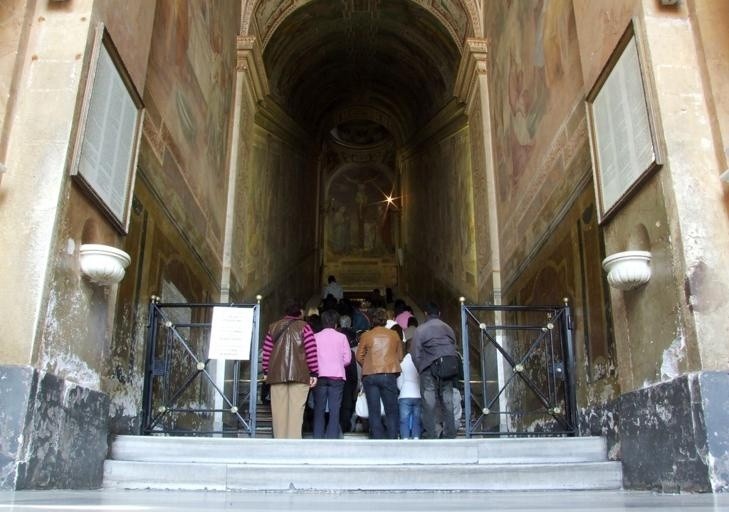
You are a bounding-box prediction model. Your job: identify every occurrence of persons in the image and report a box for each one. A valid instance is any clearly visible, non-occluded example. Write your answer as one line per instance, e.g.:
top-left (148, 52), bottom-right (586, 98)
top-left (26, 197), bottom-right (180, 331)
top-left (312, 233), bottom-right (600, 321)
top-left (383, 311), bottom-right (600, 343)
top-left (263, 274), bottom-right (463, 439)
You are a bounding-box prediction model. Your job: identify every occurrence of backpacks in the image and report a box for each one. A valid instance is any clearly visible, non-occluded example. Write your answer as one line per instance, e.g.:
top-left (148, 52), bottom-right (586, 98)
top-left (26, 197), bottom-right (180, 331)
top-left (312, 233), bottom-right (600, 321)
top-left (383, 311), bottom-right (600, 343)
top-left (433, 356), bottom-right (460, 377)
